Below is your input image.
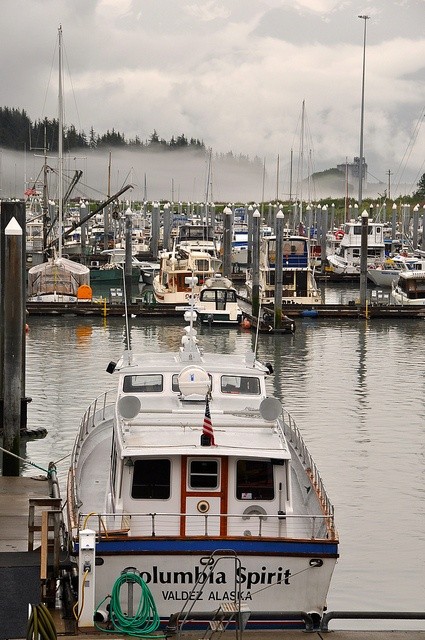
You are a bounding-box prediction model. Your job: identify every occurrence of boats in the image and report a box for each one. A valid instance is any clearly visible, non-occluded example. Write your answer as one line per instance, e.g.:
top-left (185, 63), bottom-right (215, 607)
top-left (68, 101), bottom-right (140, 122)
top-left (23, 127), bottom-right (149, 253)
top-left (327, 214), bottom-right (387, 275)
top-left (386, 213), bottom-right (424, 249)
top-left (245, 235), bottom-right (323, 305)
top-left (392, 263), bottom-right (424, 318)
top-left (66, 268), bottom-right (339, 624)
top-left (366, 249), bottom-right (424, 287)
top-left (152, 236), bottom-right (222, 303)
top-left (192, 273), bottom-right (245, 328)
top-left (24, 258), bottom-right (93, 304)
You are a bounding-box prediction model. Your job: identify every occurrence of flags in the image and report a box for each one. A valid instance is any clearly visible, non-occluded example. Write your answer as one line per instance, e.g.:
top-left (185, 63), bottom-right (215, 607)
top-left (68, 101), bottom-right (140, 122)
top-left (202, 398), bottom-right (215, 446)
